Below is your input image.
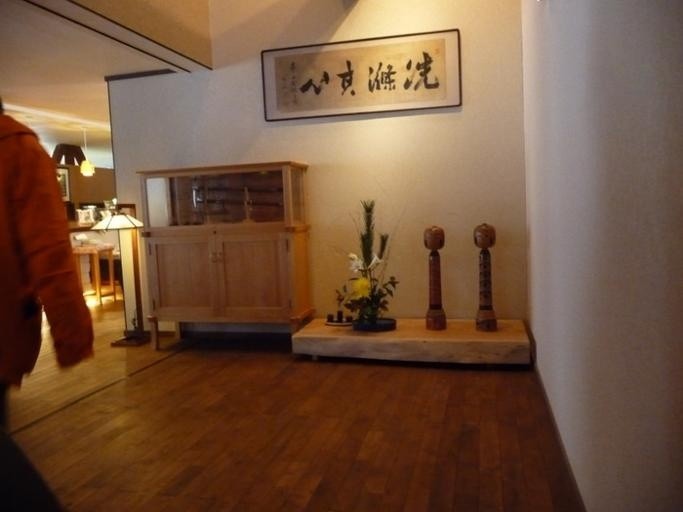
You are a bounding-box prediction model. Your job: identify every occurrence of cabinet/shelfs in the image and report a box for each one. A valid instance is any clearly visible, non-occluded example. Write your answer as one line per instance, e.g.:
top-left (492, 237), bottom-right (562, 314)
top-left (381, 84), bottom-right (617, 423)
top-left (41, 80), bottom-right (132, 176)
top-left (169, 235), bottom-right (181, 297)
top-left (135, 160), bottom-right (313, 351)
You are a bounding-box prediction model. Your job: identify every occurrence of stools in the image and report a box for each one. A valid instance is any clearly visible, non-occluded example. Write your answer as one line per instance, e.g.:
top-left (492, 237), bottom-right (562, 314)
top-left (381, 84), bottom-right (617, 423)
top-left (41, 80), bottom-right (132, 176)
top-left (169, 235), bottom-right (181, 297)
top-left (73, 245), bottom-right (116, 308)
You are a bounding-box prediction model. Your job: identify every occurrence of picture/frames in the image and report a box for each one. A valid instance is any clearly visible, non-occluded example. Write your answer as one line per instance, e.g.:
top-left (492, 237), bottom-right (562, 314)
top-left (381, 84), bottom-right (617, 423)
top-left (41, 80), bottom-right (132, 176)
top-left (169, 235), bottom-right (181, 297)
top-left (260, 29), bottom-right (462, 122)
top-left (56, 166), bottom-right (71, 202)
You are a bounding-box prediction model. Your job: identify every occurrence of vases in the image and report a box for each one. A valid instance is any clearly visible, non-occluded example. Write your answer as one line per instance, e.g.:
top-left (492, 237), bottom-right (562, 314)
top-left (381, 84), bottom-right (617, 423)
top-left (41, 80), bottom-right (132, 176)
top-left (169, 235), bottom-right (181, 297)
top-left (352, 314), bottom-right (396, 331)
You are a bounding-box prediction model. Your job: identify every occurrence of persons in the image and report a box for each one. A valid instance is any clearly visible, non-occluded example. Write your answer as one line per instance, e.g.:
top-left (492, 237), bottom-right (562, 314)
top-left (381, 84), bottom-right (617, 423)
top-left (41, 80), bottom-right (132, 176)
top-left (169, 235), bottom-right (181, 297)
top-left (2, 99), bottom-right (94, 511)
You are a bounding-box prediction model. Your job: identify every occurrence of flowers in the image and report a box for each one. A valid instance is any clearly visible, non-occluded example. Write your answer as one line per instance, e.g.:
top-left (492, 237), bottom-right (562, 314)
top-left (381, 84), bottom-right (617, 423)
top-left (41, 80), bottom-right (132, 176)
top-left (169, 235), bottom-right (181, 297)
top-left (335, 200), bottom-right (398, 323)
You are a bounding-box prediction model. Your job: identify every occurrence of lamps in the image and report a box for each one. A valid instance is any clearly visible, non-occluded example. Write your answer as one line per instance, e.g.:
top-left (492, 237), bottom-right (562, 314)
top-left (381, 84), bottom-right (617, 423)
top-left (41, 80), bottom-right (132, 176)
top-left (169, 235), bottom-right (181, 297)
top-left (91, 204), bottom-right (148, 347)
top-left (80, 129), bottom-right (95, 177)
